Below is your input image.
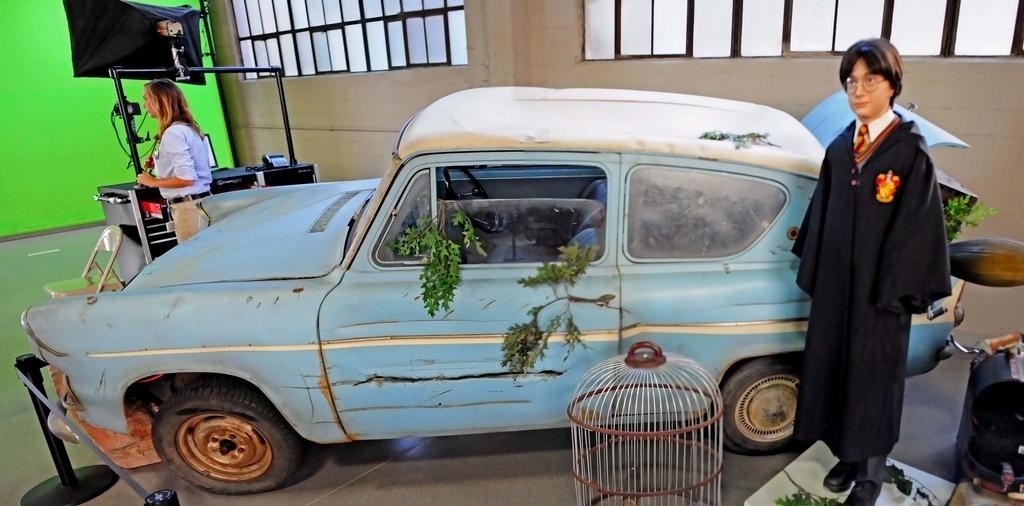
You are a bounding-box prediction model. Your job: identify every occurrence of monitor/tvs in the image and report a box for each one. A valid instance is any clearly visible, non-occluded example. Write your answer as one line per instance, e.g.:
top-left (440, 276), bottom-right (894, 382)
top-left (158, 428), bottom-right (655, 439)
top-left (202, 134), bottom-right (219, 168)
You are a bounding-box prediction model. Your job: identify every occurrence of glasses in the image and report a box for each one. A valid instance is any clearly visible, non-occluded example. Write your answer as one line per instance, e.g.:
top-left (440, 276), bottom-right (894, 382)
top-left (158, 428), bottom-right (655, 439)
top-left (844, 75), bottom-right (889, 93)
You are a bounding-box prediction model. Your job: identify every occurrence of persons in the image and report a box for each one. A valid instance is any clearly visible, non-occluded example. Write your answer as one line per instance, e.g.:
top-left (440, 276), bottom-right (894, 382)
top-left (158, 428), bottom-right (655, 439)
top-left (135, 78), bottom-right (213, 209)
top-left (783, 39), bottom-right (952, 506)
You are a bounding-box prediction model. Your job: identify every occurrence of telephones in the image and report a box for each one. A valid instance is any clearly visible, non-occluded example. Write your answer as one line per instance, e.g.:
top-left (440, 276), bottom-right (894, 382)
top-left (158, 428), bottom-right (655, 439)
top-left (262, 154), bottom-right (290, 169)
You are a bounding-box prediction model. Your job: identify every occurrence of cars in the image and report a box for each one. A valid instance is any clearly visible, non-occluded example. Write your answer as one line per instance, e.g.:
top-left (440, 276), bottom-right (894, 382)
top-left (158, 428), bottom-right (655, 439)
top-left (16, 85), bottom-right (978, 495)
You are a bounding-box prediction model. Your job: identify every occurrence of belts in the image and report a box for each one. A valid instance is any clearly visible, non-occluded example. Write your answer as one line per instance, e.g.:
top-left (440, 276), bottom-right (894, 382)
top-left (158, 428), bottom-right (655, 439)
top-left (169, 191), bottom-right (210, 205)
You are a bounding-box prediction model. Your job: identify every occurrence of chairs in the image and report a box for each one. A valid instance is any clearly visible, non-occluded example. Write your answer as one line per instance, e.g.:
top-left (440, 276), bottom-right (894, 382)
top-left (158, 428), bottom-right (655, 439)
top-left (43, 224), bottom-right (124, 300)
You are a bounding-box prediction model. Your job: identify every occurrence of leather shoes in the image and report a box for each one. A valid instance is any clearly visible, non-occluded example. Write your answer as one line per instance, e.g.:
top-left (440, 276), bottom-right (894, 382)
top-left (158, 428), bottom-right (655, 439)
top-left (825, 450), bottom-right (851, 492)
top-left (845, 476), bottom-right (884, 506)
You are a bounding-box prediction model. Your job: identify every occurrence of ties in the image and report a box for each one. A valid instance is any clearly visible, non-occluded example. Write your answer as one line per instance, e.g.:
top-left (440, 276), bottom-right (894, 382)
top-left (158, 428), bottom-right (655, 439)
top-left (853, 124), bottom-right (874, 155)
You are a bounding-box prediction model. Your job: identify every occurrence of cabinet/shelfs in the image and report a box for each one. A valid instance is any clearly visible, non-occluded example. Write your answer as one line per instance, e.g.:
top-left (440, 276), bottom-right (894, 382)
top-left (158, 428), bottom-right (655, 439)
top-left (92, 163), bottom-right (319, 285)
